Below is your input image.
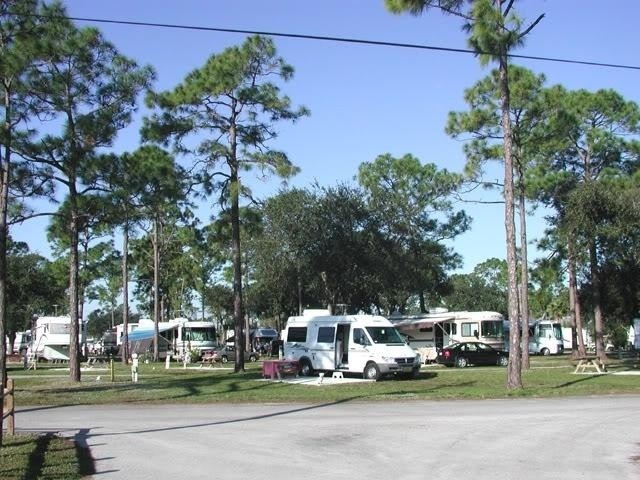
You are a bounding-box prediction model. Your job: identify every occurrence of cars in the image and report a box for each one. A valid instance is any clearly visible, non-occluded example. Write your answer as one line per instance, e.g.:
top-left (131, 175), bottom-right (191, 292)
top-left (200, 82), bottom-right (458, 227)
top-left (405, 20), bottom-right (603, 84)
top-left (437, 341), bottom-right (509, 368)
top-left (203, 345), bottom-right (260, 364)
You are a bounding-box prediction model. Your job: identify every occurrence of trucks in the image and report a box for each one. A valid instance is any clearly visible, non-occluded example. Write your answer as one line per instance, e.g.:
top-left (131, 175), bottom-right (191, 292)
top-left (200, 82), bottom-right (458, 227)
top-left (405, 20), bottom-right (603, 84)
top-left (6, 332), bottom-right (32, 356)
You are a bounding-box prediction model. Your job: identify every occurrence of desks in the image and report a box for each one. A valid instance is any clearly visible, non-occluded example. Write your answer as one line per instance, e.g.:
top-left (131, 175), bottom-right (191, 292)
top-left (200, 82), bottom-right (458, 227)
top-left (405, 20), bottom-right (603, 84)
top-left (199, 357), bottom-right (224, 368)
top-left (85, 357), bottom-right (109, 368)
top-left (574, 355), bottom-right (604, 373)
top-left (257, 360), bottom-right (299, 379)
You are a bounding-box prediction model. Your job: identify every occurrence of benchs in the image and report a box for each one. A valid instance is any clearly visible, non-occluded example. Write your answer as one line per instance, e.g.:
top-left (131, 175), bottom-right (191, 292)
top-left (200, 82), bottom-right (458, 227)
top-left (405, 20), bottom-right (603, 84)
top-left (197, 361), bottom-right (213, 364)
top-left (80, 362), bottom-right (95, 365)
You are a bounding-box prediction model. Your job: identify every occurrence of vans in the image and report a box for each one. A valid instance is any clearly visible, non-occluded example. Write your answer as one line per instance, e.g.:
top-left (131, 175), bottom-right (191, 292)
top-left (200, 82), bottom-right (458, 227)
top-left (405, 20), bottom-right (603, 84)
top-left (283, 314), bottom-right (422, 381)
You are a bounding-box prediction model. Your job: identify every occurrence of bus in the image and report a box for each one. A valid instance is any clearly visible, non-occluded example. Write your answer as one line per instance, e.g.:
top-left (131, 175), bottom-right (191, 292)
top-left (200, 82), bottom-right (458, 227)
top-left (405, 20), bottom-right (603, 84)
top-left (385, 311), bottom-right (504, 363)
top-left (226, 328), bottom-right (279, 355)
top-left (504, 319), bottom-right (564, 356)
top-left (115, 321), bottom-right (217, 362)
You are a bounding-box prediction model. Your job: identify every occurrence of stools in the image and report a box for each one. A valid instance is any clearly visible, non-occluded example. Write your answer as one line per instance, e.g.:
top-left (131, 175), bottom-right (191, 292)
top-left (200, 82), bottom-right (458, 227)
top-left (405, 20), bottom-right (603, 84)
top-left (332, 372), bottom-right (343, 382)
